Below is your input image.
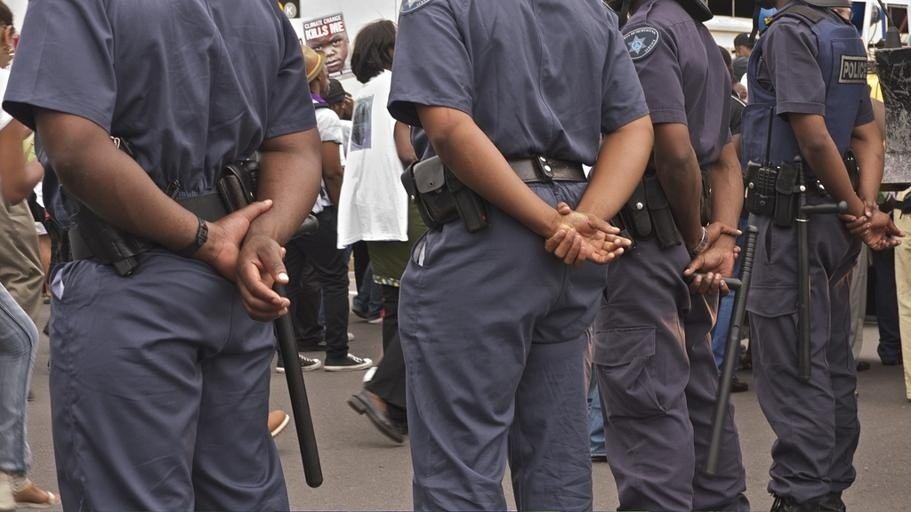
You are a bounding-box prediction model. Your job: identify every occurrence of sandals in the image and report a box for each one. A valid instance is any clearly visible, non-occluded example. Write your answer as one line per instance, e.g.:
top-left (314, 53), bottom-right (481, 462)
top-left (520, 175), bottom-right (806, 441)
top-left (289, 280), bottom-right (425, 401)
top-left (1, 477), bottom-right (60, 509)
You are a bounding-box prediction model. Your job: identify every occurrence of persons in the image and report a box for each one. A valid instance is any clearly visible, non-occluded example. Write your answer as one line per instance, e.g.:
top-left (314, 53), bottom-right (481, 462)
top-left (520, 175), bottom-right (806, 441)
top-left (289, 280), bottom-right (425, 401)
top-left (335, 20), bottom-right (428, 445)
top-left (591, 383), bottom-right (609, 459)
top-left (848, 223), bottom-right (903, 371)
top-left (0, 6), bottom-right (57, 509)
top-left (274, 42), bottom-right (375, 373)
top-left (592, 0), bottom-right (751, 512)
top-left (306, 22), bottom-right (347, 73)
top-left (741, 1), bottom-right (903, 511)
top-left (389, 2), bottom-right (654, 512)
top-left (3, 1), bottom-right (324, 512)
top-left (712, 28), bottom-right (758, 394)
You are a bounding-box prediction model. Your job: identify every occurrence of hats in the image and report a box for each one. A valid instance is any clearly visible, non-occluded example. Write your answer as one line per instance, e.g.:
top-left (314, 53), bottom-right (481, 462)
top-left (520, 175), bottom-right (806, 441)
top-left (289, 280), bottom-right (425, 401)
top-left (301, 44), bottom-right (326, 83)
top-left (734, 33), bottom-right (757, 46)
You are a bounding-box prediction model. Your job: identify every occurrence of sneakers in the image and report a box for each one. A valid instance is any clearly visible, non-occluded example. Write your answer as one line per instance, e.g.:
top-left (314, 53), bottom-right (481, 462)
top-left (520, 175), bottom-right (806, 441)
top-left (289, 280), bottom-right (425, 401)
top-left (369, 311), bottom-right (385, 323)
top-left (323, 353), bottom-right (372, 371)
top-left (352, 298), bottom-right (369, 318)
top-left (346, 331), bottom-right (354, 340)
top-left (276, 355), bottom-right (321, 372)
top-left (364, 367), bottom-right (378, 382)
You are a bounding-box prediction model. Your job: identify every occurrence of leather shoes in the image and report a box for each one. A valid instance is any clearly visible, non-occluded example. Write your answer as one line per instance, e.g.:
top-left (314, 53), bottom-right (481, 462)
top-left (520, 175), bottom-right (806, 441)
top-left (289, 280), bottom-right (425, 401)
top-left (857, 360), bottom-right (870, 371)
top-left (346, 391), bottom-right (404, 443)
top-left (770, 497), bottom-right (818, 511)
top-left (270, 410), bottom-right (289, 434)
top-left (729, 378), bottom-right (749, 391)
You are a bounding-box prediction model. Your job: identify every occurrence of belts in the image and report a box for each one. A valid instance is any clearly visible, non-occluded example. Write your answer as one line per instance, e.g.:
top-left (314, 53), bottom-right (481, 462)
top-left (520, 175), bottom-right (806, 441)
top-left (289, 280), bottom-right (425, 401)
top-left (507, 158), bottom-right (588, 184)
top-left (67, 186), bottom-right (230, 260)
